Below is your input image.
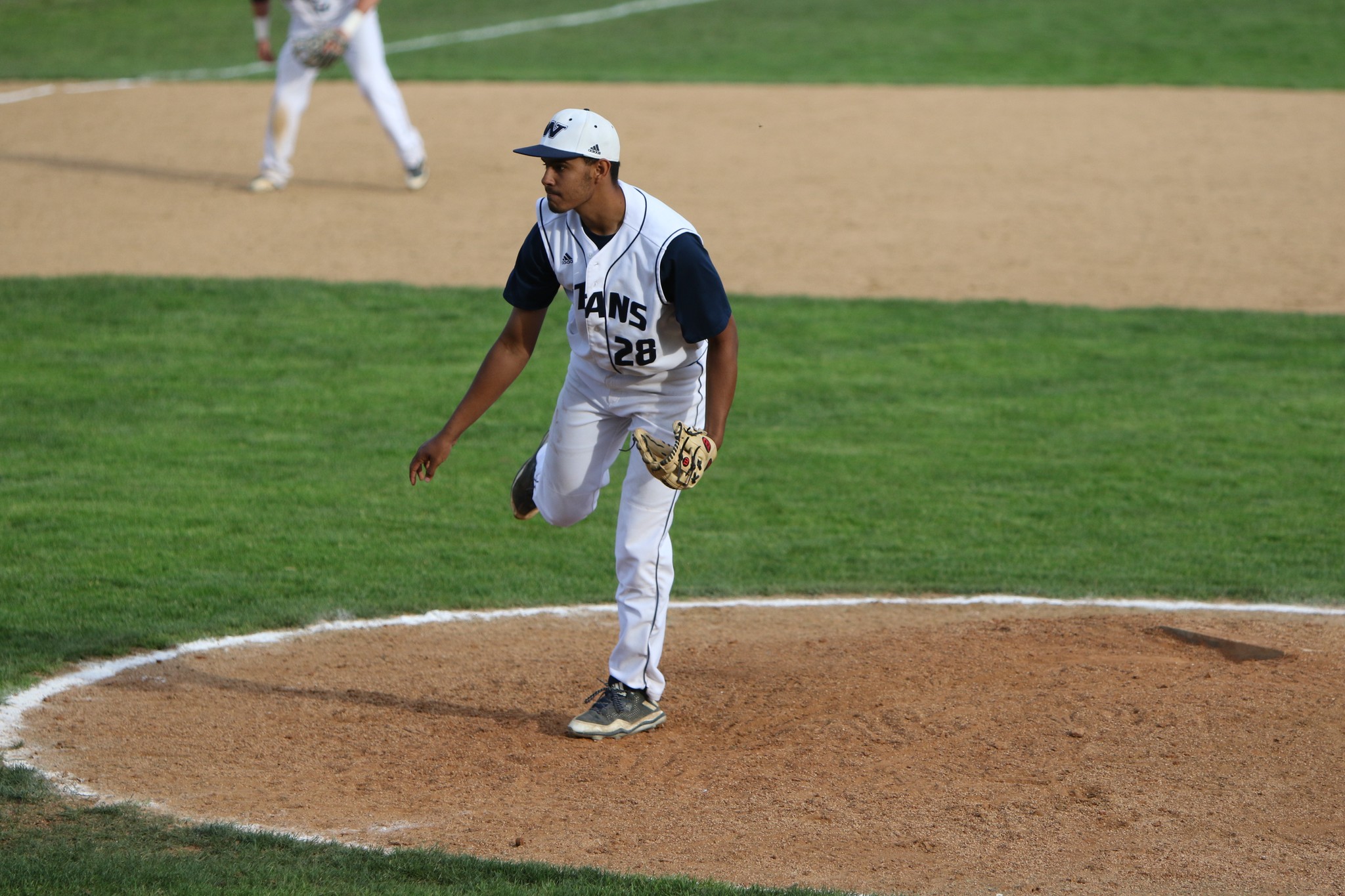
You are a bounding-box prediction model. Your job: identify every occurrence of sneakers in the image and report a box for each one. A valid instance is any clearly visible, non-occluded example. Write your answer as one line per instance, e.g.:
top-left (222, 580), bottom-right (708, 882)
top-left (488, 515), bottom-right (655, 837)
top-left (567, 676), bottom-right (667, 740)
top-left (510, 433), bottom-right (551, 520)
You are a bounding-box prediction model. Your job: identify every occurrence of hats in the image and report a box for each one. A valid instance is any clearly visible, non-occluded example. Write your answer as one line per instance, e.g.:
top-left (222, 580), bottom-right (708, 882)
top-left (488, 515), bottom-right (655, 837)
top-left (513, 108), bottom-right (621, 162)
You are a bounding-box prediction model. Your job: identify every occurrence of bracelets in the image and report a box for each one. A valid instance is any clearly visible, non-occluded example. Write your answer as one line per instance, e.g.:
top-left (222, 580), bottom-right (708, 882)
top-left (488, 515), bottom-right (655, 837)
top-left (254, 19), bottom-right (270, 42)
top-left (339, 7), bottom-right (364, 37)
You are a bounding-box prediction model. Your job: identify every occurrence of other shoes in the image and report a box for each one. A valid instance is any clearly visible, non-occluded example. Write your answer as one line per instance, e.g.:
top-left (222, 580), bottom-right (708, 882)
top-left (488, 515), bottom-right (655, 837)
top-left (407, 161), bottom-right (429, 191)
top-left (247, 177), bottom-right (273, 194)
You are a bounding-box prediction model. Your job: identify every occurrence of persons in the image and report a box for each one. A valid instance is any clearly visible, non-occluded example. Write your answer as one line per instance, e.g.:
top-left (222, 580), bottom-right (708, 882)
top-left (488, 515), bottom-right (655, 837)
top-left (244, 0), bottom-right (429, 192)
top-left (408, 106), bottom-right (739, 740)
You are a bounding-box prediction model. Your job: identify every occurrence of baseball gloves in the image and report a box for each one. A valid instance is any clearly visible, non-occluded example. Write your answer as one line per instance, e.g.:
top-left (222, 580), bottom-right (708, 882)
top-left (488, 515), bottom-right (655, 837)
top-left (288, 28), bottom-right (347, 67)
top-left (633, 422), bottom-right (718, 490)
